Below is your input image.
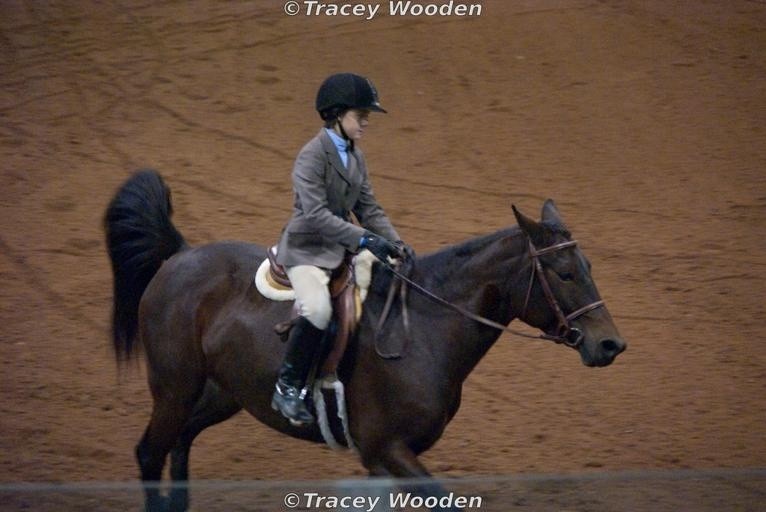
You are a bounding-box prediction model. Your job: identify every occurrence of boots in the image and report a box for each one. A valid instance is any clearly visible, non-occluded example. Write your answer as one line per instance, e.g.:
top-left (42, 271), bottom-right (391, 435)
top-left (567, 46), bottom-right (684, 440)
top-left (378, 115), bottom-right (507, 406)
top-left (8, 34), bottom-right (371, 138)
top-left (270, 316), bottom-right (325, 428)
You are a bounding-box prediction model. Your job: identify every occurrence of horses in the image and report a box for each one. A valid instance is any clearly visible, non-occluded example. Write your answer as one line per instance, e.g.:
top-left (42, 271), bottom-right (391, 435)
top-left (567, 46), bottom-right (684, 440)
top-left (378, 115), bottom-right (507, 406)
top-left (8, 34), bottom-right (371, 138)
top-left (99, 166), bottom-right (632, 511)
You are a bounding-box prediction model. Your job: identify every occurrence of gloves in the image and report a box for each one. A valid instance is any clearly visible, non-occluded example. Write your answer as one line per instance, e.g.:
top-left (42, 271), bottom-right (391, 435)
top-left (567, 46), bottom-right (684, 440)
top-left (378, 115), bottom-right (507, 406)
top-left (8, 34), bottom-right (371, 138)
top-left (394, 240), bottom-right (416, 263)
top-left (362, 232), bottom-right (400, 264)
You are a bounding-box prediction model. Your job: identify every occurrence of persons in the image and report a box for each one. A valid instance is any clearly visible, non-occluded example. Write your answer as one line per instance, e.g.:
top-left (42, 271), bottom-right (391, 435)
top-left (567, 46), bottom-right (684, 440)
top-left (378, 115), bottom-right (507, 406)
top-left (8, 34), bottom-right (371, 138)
top-left (266, 71), bottom-right (414, 426)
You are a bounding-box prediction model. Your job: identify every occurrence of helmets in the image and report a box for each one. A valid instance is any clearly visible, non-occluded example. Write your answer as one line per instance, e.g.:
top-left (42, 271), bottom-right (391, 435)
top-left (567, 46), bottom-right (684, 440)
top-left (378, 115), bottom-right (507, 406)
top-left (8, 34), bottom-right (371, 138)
top-left (315, 73), bottom-right (388, 120)
